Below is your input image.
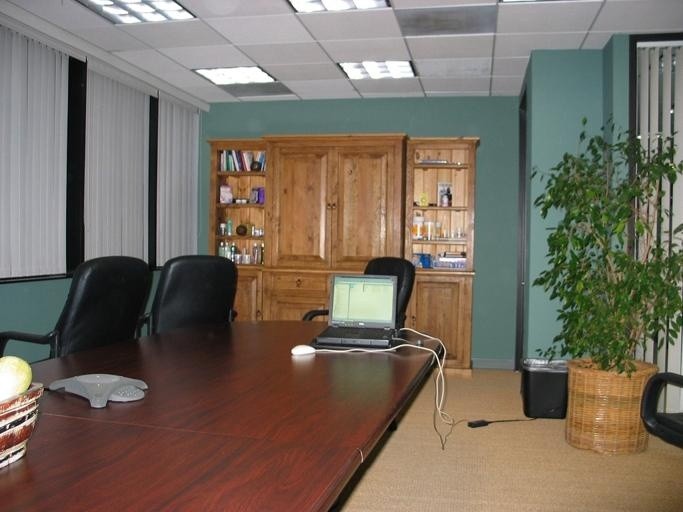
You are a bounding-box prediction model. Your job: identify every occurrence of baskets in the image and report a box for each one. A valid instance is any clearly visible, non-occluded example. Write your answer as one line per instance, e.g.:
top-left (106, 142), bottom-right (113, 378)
top-left (565, 358), bottom-right (660, 456)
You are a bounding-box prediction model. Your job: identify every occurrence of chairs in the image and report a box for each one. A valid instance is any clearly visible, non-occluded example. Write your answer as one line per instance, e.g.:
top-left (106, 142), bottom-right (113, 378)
top-left (302, 257), bottom-right (414, 328)
top-left (1, 256), bottom-right (240, 366)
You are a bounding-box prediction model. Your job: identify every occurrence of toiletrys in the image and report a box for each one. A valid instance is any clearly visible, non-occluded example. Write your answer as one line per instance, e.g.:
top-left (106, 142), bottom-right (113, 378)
top-left (217, 219), bottom-right (265, 267)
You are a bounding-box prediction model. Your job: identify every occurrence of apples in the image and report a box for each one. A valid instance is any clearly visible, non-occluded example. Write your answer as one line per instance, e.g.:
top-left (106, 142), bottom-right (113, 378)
top-left (0, 356), bottom-right (32, 399)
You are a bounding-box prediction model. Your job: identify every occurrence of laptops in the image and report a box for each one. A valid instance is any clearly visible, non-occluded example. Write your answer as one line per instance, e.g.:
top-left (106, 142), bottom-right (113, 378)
top-left (315, 275), bottom-right (398, 346)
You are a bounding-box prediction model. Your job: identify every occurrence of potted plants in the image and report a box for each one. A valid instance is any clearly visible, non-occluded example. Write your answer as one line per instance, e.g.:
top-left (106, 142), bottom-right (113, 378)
top-left (524, 114), bottom-right (683, 456)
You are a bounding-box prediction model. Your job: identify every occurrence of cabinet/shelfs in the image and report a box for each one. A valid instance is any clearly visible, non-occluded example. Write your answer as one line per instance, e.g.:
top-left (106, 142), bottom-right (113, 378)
top-left (403, 137), bottom-right (480, 370)
top-left (203, 138), bottom-right (271, 321)
top-left (261, 134), bottom-right (407, 319)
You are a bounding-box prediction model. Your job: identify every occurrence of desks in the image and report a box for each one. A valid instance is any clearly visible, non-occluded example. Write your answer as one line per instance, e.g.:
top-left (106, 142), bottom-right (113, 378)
top-left (0, 318), bottom-right (443, 510)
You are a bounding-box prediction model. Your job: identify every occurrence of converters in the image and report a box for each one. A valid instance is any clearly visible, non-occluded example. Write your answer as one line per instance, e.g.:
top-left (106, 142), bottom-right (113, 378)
top-left (290, 344), bottom-right (314, 356)
top-left (468, 420), bottom-right (488, 428)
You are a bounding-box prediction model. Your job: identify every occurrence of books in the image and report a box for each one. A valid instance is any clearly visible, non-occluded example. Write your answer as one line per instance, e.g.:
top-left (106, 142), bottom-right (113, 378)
top-left (219, 149), bottom-right (265, 172)
top-left (431, 251), bottom-right (465, 268)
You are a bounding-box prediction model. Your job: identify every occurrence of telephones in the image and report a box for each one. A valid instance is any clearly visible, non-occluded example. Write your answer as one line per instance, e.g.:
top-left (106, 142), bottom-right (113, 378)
top-left (50, 375), bottom-right (149, 408)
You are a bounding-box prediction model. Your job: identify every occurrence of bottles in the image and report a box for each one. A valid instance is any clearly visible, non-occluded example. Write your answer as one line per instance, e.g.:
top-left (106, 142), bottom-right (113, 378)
top-left (220, 219), bottom-right (232, 237)
top-left (412, 216), bottom-right (442, 242)
top-left (219, 241), bottom-right (265, 265)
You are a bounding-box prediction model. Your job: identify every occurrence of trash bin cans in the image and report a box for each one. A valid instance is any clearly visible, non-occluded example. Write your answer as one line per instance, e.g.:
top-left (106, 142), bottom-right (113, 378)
top-left (522, 359), bottom-right (568, 419)
